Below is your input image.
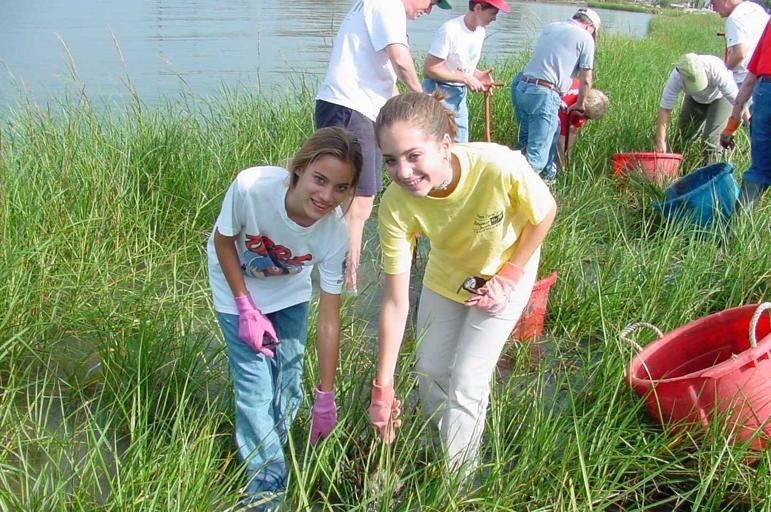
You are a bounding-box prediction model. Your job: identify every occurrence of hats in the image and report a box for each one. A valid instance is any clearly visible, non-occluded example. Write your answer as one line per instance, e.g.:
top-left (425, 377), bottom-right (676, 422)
top-left (576, 6), bottom-right (600, 43)
top-left (435, 0), bottom-right (452, 9)
top-left (677, 52), bottom-right (708, 93)
top-left (471, 0), bottom-right (511, 13)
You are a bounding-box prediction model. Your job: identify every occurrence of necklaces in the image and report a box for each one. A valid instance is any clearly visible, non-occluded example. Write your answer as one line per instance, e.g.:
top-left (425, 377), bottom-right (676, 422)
top-left (432, 159), bottom-right (454, 191)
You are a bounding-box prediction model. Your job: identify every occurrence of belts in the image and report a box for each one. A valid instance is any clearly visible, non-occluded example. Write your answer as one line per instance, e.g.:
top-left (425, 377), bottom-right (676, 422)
top-left (519, 75), bottom-right (560, 93)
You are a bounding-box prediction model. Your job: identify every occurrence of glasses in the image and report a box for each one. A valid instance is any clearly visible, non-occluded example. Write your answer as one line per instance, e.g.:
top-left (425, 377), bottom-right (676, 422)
top-left (456, 277), bottom-right (487, 296)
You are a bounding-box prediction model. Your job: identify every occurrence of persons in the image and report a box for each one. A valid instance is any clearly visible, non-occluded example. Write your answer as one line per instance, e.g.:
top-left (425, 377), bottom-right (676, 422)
top-left (511, 6), bottom-right (602, 179)
top-left (720, 15), bottom-right (771, 211)
top-left (511, 71), bottom-right (610, 186)
top-left (653, 50), bottom-right (751, 172)
top-left (313, 0), bottom-right (453, 294)
top-left (421, 0), bottom-right (513, 145)
top-left (205, 124), bottom-right (366, 511)
top-left (710, 1), bottom-right (770, 109)
top-left (366, 87), bottom-right (558, 493)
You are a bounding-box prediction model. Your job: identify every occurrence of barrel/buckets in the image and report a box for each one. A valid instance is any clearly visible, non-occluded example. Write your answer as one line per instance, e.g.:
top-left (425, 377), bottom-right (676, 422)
top-left (618, 298), bottom-right (770, 468)
top-left (507, 269), bottom-right (559, 342)
top-left (609, 150), bottom-right (685, 188)
top-left (650, 157), bottom-right (742, 244)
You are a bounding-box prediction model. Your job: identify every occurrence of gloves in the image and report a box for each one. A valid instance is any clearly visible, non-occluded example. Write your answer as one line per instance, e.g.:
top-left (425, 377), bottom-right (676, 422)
top-left (368, 378), bottom-right (401, 444)
top-left (465, 262), bottom-right (524, 316)
top-left (720, 116), bottom-right (740, 149)
top-left (310, 385), bottom-right (336, 446)
top-left (233, 292), bottom-right (278, 358)
